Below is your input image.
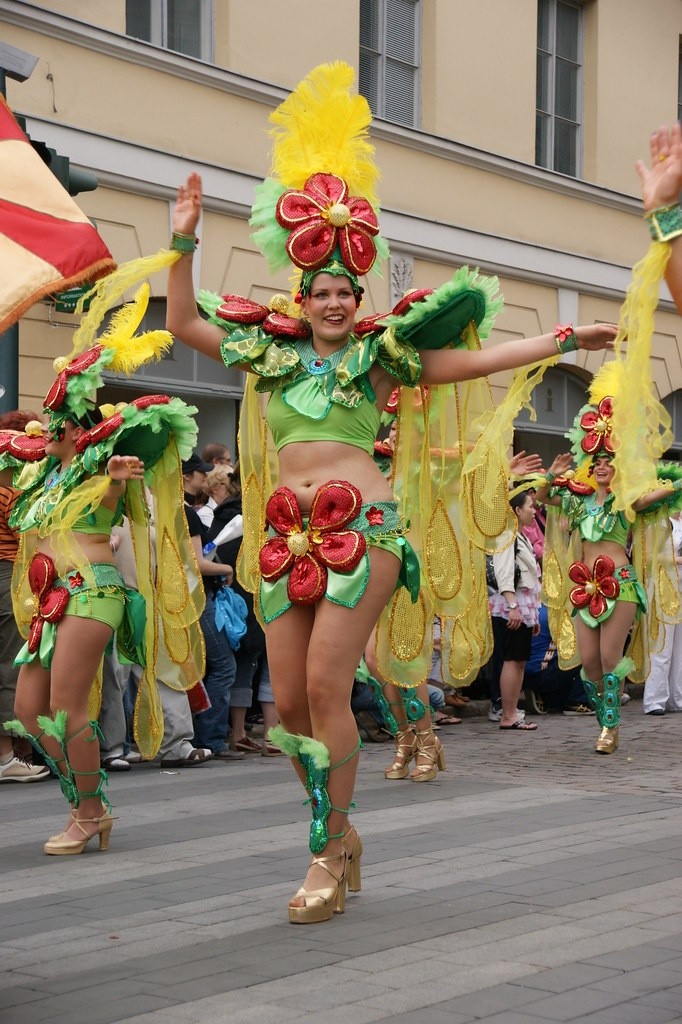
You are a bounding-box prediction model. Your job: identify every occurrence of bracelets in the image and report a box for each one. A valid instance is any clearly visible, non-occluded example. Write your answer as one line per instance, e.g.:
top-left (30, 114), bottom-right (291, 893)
top-left (545, 471), bottom-right (556, 482)
top-left (104, 467), bottom-right (122, 485)
top-left (508, 603), bottom-right (517, 609)
top-left (169, 231), bottom-right (199, 254)
top-left (673, 478), bottom-right (682, 491)
top-left (552, 323), bottom-right (579, 354)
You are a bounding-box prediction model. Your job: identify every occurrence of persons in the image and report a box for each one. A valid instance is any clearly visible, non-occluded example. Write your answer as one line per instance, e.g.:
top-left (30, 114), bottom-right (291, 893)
top-left (0, 410), bottom-right (50, 783)
top-left (8, 398), bottom-right (200, 855)
top-left (95, 443), bottom-right (287, 772)
top-left (366, 418), bottom-right (542, 783)
top-left (610, 124), bottom-right (682, 524)
top-left (350, 468), bottom-right (682, 742)
top-left (507, 451), bottom-right (682, 754)
top-left (54, 172), bottom-right (628, 923)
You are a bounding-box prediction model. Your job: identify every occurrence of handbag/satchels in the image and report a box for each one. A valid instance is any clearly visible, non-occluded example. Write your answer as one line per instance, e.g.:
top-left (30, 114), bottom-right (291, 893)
top-left (486, 534), bottom-right (521, 591)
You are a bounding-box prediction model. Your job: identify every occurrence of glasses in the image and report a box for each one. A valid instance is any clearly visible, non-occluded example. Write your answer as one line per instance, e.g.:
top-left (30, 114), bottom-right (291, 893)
top-left (219, 455), bottom-right (231, 462)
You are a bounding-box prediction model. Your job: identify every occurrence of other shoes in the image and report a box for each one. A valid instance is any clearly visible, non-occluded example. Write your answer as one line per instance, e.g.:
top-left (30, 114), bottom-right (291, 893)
top-left (0, 692), bottom-right (666, 781)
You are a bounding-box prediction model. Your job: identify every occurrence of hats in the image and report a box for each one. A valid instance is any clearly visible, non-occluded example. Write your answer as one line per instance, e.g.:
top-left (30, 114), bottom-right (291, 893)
top-left (181, 453), bottom-right (215, 471)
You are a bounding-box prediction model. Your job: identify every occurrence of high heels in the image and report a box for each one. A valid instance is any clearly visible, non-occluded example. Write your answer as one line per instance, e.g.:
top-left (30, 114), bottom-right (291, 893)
top-left (288, 844), bottom-right (346, 923)
top-left (44, 801), bottom-right (120, 854)
top-left (341, 825), bottom-right (363, 892)
